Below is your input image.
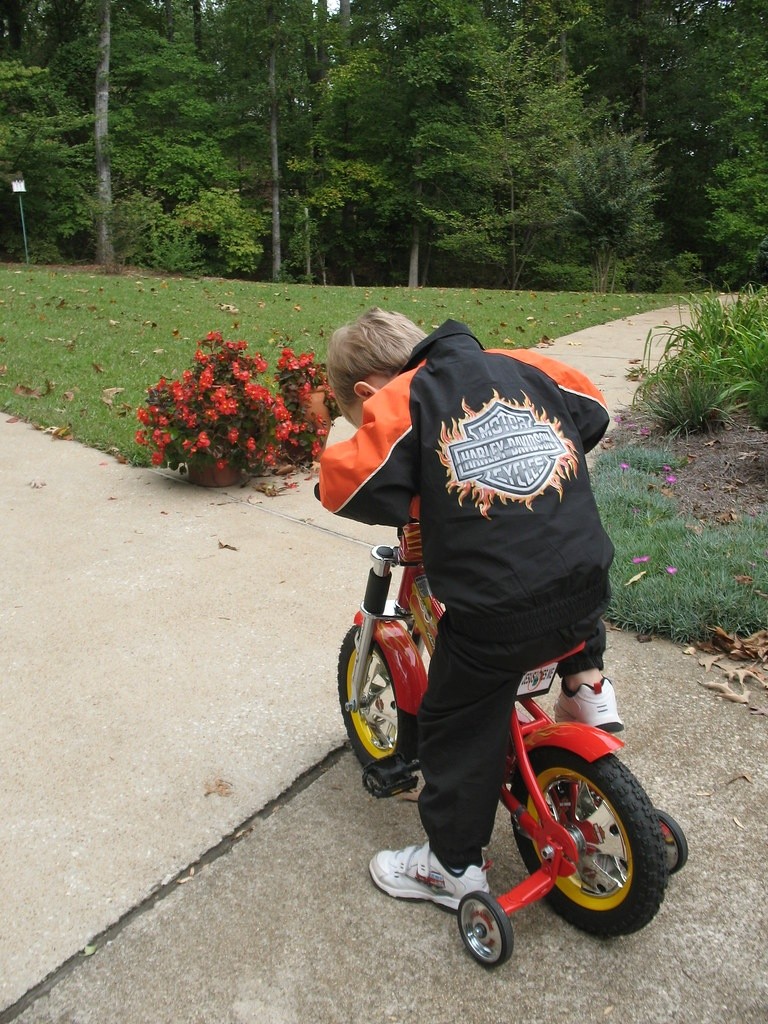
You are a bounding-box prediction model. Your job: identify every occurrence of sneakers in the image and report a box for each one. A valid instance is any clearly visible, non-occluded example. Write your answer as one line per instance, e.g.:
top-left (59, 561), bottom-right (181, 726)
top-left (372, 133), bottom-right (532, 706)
top-left (553, 677), bottom-right (625, 732)
top-left (369, 840), bottom-right (490, 911)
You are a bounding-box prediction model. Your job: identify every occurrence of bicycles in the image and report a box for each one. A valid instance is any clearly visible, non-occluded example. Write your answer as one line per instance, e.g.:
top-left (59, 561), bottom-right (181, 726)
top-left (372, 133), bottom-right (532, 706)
top-left (312, 476), bottom-right (691, 970)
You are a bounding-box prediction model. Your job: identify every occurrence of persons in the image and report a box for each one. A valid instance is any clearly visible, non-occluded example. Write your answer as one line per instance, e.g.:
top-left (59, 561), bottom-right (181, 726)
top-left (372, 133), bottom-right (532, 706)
top-left (319, 306), bottom-right (627, 911)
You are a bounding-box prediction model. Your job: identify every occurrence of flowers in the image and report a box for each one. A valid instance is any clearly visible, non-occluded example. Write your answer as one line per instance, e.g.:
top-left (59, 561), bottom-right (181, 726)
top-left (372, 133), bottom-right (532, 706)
top-left (136, 329), bottom-right (294, 469)
top-left (276, 347), bottom-right (342, 466)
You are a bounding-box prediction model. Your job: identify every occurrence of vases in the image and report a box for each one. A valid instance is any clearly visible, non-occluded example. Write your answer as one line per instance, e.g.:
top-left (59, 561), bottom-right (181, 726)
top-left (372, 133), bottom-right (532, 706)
top-left (297, 390), bottom-right (331, 463)
top-left (185, 459), bottom-right (242, 488)
